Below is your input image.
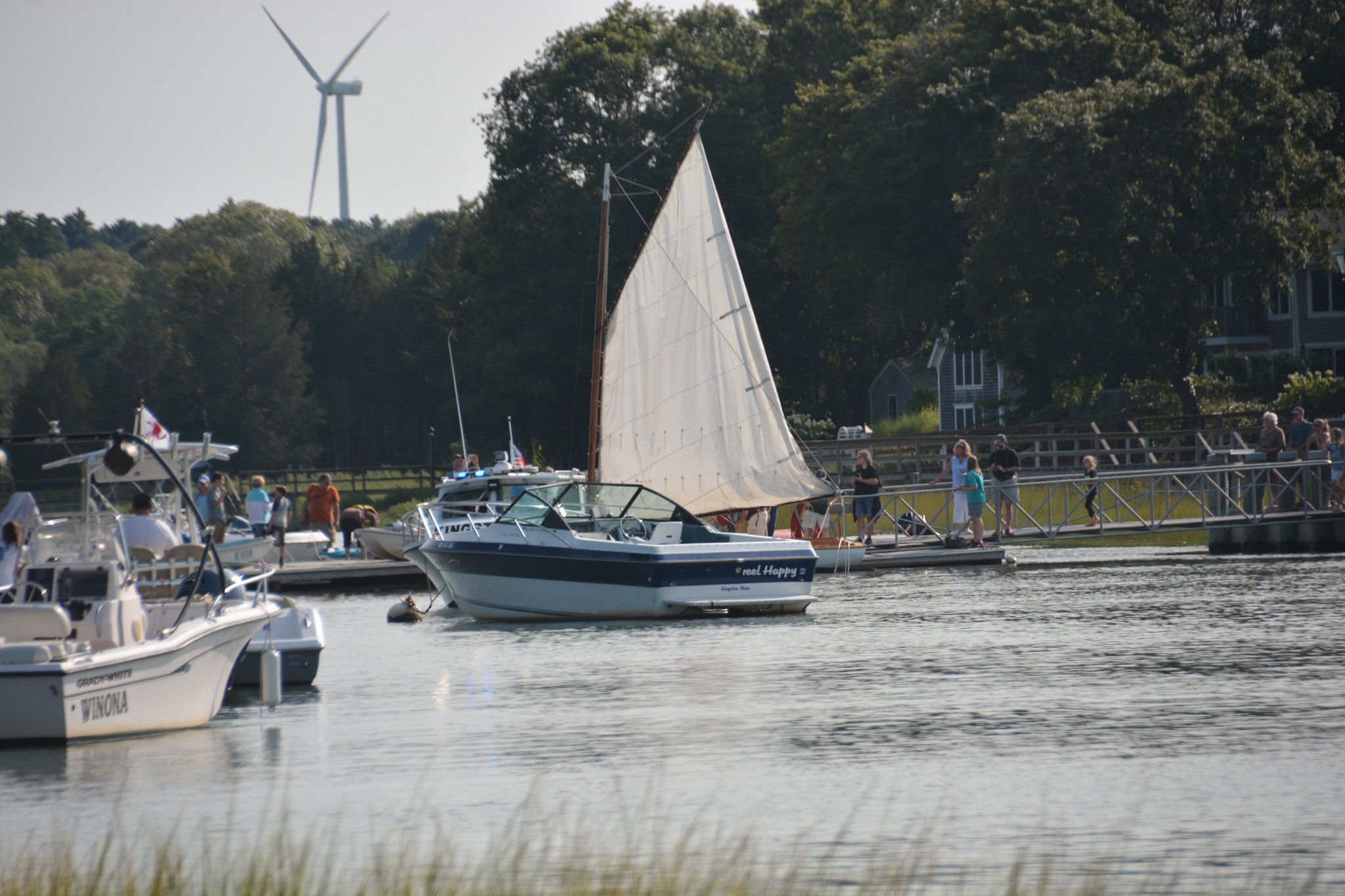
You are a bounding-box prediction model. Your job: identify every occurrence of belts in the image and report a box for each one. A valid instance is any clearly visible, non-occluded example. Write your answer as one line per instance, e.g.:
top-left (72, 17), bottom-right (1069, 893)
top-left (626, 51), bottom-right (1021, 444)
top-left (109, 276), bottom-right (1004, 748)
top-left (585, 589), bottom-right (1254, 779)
top-left (997, 476), bottom-right (1013, 481)
top-left (751, 509), bottom-right (766, 516)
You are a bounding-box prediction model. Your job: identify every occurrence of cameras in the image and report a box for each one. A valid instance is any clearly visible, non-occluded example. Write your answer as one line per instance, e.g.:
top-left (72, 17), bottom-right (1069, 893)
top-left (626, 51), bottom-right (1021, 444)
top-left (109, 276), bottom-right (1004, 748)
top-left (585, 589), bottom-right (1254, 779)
top-left (470, 455), bottom-right (475, 460)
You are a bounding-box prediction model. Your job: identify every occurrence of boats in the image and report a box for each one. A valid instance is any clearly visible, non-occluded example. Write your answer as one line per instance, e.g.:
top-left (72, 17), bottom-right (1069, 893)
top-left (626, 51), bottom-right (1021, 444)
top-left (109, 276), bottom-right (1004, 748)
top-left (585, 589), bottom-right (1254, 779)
top-left (0, 93), bottom-right (868, 751)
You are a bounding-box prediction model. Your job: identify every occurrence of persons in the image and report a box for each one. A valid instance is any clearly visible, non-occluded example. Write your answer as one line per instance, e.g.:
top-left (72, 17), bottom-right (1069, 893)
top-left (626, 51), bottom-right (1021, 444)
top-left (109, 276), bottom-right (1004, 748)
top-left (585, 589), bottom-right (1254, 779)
top-left (447, 453), bottom-right (480, 480)
top-left (711, 507), bottom-right (771, 536)
top-left (112, 492), bottom-right (180, 559)
top-left (801, 469), bottom-right (839, 538)
top-left (0, 521), bottom-right (28, 586)
top-left (987, 434), bottom-right (1021, 536)
top-left (959, 455), bottom-right (985, 548)
top-left (1079, 455), bottom-right (1100, 527)
top-left (510, 486), bottom-right (541, 504)
top-left (850, 450), bottom-right (882, 545)
top-left (928, 439), bottom-right (974, 536)
top-left (192, 472), bottom-right (380, 566)
top-left (1254, 406), bottom-right (1345, 514)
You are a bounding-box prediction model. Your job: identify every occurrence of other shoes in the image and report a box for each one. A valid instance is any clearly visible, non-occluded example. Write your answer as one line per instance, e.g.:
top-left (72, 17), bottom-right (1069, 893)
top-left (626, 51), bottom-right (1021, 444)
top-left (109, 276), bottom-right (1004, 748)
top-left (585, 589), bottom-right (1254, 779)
top-left (857, 539), bottom-right (862, 542)
top-left (1092, 518), bottom-right (1100, 524)
top-left (1005, 529), bottom-right (1014, 535)
top-left (968, 539), bottom-right (978, 546)
top-left (1085, 523), bottom-right (1095, 527)
top-left (949, 530), bottom-right (958, 536)
top-left (865, 538), bottom-right (872, 544)
top-left (971, 542), bottom-right (985, 548)
top-left (992, 529), bottom-right (1003, 535)
top-left (966, 529), bottom-right (973, 534)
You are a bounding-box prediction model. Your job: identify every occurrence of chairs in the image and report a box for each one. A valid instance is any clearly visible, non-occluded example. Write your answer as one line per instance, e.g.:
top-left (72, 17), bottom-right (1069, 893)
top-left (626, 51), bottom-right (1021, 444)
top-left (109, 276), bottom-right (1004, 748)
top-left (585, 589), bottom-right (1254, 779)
top-left (626, 522), bottom-right (683, 548)
top-left (0, 604), bottom-right (71, 662)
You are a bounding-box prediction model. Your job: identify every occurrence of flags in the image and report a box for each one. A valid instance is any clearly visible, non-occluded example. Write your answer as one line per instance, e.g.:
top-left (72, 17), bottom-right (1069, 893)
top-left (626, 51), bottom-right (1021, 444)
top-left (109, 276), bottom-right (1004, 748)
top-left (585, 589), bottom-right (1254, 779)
top-left (513, 444), bottom-right (525, 468)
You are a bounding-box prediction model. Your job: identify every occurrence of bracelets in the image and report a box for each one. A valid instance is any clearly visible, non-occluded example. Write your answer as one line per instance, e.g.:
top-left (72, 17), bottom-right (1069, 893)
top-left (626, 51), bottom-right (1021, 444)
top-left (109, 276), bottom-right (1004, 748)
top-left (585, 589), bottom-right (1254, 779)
top-left (766, 510), bottom-right (770, 513)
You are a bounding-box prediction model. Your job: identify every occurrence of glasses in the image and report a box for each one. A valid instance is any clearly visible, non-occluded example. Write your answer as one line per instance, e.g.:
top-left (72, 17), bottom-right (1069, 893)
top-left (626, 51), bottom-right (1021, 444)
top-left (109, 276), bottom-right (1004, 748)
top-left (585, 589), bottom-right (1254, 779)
top-left (995, 441), bottom-right (1000, 445)
top-left (956, 445), bottom-right (964, 449)
top-left (1312, 423), bottom-right (1319, 427)
top-left (856, 456), bottom-right (862, 460)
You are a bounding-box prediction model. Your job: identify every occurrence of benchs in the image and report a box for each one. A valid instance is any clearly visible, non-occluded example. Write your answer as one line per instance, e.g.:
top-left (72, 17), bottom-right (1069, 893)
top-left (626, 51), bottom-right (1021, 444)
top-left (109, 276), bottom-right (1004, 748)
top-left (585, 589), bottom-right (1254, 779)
top-left (135, 543), bottom-right (215, 601)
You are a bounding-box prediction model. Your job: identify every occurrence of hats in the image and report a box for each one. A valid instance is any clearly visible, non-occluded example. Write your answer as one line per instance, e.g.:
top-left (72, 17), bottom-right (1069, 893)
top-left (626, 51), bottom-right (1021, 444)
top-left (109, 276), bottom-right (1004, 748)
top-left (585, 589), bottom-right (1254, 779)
top-left (1292, 407), bottom-right (1303, 414)
top-left (452, 454), bottom-right (465, 460)
top-left (198, 475), bottom-right (210, 484)
top-left (816, 469), bottom-right (826, 481)
